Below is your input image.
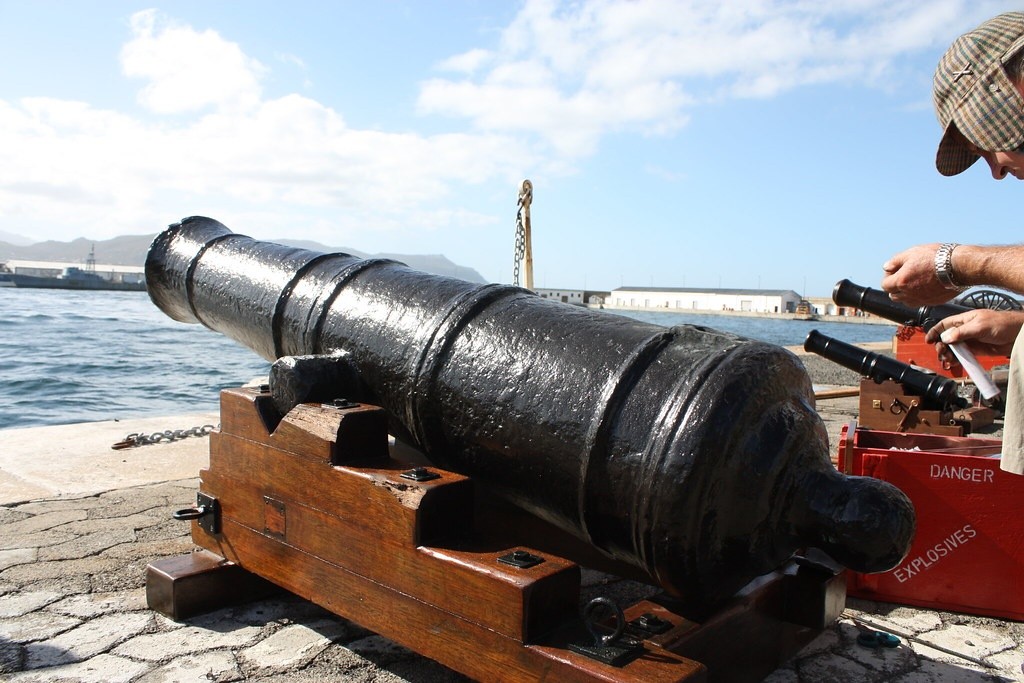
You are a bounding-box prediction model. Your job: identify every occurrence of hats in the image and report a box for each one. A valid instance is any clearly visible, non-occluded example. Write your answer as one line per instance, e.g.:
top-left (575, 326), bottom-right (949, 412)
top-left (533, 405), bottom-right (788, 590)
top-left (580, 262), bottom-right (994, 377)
top-left (932, 11), bottom-right (1024, 176)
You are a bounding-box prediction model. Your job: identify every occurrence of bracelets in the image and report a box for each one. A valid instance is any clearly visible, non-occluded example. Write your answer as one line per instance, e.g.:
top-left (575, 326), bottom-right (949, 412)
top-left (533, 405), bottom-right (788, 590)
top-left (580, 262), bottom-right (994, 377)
top-left (935, 242), bottom-right (973, 291)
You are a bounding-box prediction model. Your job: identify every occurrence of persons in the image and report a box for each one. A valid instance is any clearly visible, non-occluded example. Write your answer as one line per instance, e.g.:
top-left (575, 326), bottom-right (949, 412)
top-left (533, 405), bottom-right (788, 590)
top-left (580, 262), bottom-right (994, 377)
top-left (882, 13), bottom-right (1024, 475)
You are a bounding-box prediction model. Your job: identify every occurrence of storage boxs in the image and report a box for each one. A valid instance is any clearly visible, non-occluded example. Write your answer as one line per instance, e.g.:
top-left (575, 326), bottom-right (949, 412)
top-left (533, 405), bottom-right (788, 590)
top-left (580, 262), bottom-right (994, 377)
top-left (838, 424), bottom-right (1024, 623)
top-left (896, 325), bottom-right (1012, 382)
